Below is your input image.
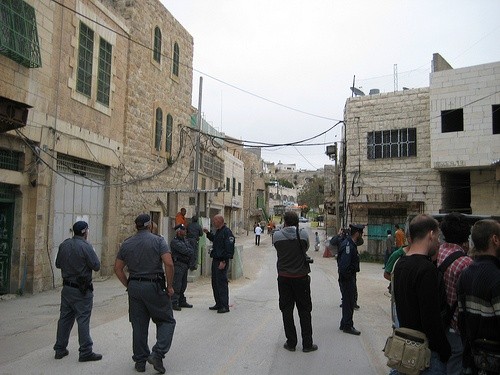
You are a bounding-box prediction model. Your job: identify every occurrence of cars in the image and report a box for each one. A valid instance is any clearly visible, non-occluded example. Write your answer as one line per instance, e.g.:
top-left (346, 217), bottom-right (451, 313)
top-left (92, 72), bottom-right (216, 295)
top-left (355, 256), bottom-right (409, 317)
top-left (298, 216), bottom-right (309, 223)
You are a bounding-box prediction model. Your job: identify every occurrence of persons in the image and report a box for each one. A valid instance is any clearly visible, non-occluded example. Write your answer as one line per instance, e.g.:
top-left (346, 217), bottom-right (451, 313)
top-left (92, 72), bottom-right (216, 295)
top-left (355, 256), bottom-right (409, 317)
top-left (114, 214), bottom-right (176, 374)
top-left (382, 230), bottom-right (394, 269)
top-left (176, 208), bottom-right (188, 226)
top-left (268, 218), bottom-right (273, 231)
top-left (400, 227), bottom-right (408, 246)
top-left (395, 224), bottom-right (405, 249)
top-left (330, 228), bottom-right (364, 309)
top-left (273, 212), bottom-right (318, 352)
top-left (260, 219), bottom-right (266, 233)
top-left (170, 224), bottom-right (193, 310)
top-left (315, 232), bottom-right (320, 251)
top-left (384, 212), bottom-right (500, 375)
top-left (203, 214), bottom-right (235, 313)
top-left (255, 224), bottom-right (262, 245)
top-left (53, 221), bottom-right (102, 362)
top-left (338, 223), bottom-right (365, 335)
top-left (271, 226), bottom-right (278, 246)
top-left (186, 216), bottom-right (203, 270)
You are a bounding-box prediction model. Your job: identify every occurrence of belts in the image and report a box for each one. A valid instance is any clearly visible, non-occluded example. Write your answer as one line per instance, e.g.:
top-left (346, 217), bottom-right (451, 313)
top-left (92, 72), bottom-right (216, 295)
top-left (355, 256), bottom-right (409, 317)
top-left (130, 277), bottom-right (154, 282)
top-left (64, 281), bottom-right (80, 289)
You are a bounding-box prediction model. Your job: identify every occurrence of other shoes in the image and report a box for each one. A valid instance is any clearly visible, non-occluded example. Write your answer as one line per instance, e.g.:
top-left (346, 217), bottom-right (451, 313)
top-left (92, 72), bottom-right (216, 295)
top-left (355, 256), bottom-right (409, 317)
top-left (147, 351), bottom-right (166, 374)
top-left (173, 303), bottom-right (193, 311)
top-left (55, 349), bottom-right (69, 359)
top-left (302, 344), bottom-right (318, 352)
top-left (339, 325), bottom-right (361, 336)
top-left (284, 343), bottom-right (296, 351)
top-left (79, 352), bottom-right (102, 362)
top-left (209, 304), bottom-right (229, 313)
top-left (135, 361), bottom-right (146, 372)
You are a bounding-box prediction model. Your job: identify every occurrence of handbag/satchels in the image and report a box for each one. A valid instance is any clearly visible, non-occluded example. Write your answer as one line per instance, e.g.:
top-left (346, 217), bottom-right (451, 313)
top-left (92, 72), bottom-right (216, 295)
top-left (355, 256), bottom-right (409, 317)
top-left (381, 328), bottom-right (432, 375)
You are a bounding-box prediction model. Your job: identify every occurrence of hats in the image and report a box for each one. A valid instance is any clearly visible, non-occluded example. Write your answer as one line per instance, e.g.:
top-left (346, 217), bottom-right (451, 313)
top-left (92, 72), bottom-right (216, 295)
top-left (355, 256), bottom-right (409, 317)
top-left (135, 214), bottom-right (150, 226)
top-left (73, 221), bottom-right (88, 235)
top-left (349, 224), bottom-right (365, 231)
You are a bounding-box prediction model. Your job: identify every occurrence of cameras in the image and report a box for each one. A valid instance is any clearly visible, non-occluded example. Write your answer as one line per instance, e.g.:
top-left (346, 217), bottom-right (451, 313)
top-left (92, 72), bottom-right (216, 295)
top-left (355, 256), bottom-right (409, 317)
top-left (303, 255), bottom-right (313, 265)
